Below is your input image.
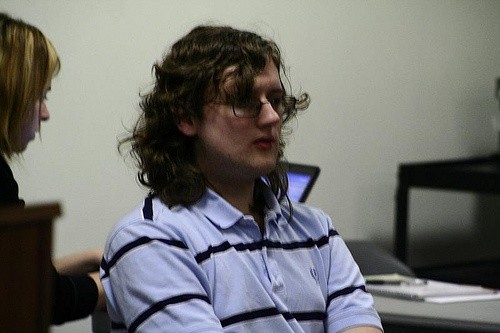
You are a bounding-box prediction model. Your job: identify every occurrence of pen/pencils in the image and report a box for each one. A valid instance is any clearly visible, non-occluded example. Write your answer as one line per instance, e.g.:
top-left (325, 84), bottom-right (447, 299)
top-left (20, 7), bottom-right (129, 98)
top-left (365, 279), bottom-right (427, 285)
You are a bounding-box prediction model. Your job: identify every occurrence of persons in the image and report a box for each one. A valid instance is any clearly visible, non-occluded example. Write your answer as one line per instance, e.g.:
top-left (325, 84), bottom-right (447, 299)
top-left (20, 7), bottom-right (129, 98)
top-left (0, 13), bottom-right (107, 333)
top-left (99, 25), bottom-right (385, 333)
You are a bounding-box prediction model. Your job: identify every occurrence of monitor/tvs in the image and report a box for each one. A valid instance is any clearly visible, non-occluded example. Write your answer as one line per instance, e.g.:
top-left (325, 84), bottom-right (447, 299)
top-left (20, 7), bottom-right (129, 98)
top-left (277, 162), bottom-right (321, 203)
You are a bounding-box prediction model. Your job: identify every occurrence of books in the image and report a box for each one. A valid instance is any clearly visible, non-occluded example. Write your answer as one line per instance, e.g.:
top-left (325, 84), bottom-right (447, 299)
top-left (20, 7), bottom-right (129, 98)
top-left (363, 273), bottom-right (500, 303)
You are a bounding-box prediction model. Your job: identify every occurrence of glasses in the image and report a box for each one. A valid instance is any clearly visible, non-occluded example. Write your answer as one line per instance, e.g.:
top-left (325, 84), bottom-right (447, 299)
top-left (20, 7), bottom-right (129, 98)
top-left (209, 95), bottom-right (300, 119)
